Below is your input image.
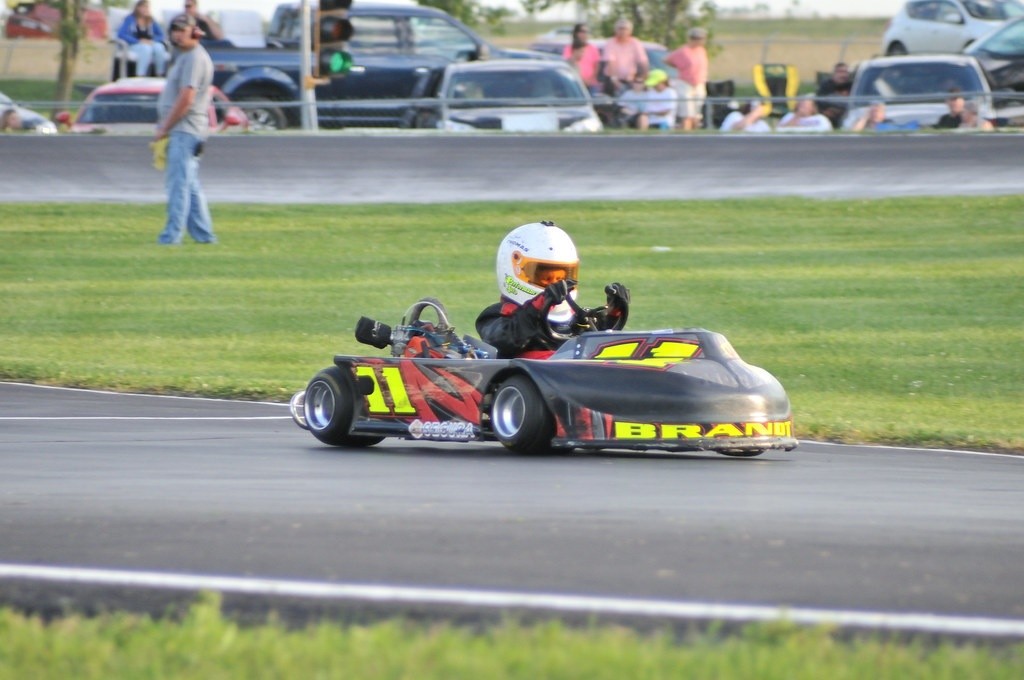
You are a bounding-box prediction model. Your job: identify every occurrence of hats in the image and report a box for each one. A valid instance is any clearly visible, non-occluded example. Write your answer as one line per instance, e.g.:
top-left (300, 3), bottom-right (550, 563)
top-left (170, 14), bottom-right (197, 30)
top-left (644, 69), bottom-right (668, 87)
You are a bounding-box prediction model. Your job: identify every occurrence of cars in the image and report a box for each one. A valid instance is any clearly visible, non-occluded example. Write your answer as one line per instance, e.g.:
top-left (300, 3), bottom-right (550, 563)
top-left (962, 15), bottom-right (1024, 92)
top-left (884, 0), bottom-right (1024, 56)
top-left (531, 27), bottom-right (680, 80)
top-left (838, 55), bottom-right (994, 131)
top-left (55, 76), bottom-right (249, 133)
top-left (433, 58), bottom-right (605, 134)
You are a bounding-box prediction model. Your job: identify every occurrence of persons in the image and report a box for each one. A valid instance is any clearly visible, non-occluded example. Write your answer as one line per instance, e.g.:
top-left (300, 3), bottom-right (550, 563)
top-left (183, 0), bottom-right (224, 41)
top-left (154, 12), bottom-right (216, 242)
top-left (4, 110), bottom-right (47, 129)
top-left (603, 15), bottom-right (649, 95)
top-left (932, 87), bottom-right (965, 128)
top-left (111, 0), bottom-right (166, 79)
top-left (616, 70), bottom-right (677, 131)
top-left (817, 61), bottom-right (853, 124)
top-left (777, 99), bottom-right (832, 131)
top-left (959, 102), bottom-right (994, 129)
top-left (474, 220), bottom-right (631, 361)
top-left (563, 24), bottom-right (602, 96)
top-left (719, 100), bottom-right (773, 132)
top-left (663, 27), bottom-right (709, 130)
top-left (851, 101), bottom-right (895, 131)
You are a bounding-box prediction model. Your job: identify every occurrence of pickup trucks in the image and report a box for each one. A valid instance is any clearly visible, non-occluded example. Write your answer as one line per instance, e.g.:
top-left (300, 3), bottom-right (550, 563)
top-left (173, 4), bottom-right (564, 129)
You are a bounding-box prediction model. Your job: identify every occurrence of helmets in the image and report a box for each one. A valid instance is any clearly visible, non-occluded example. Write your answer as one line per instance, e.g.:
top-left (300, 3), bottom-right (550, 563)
top-left (496, 221), bottom-right (580, 323)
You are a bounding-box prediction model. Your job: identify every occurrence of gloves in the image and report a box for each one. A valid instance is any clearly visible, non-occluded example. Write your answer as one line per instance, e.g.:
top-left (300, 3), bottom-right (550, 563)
top-left (605, 283), bottom-right (628, 309)
top-left (545, 279), bottom-right (577, 305)
